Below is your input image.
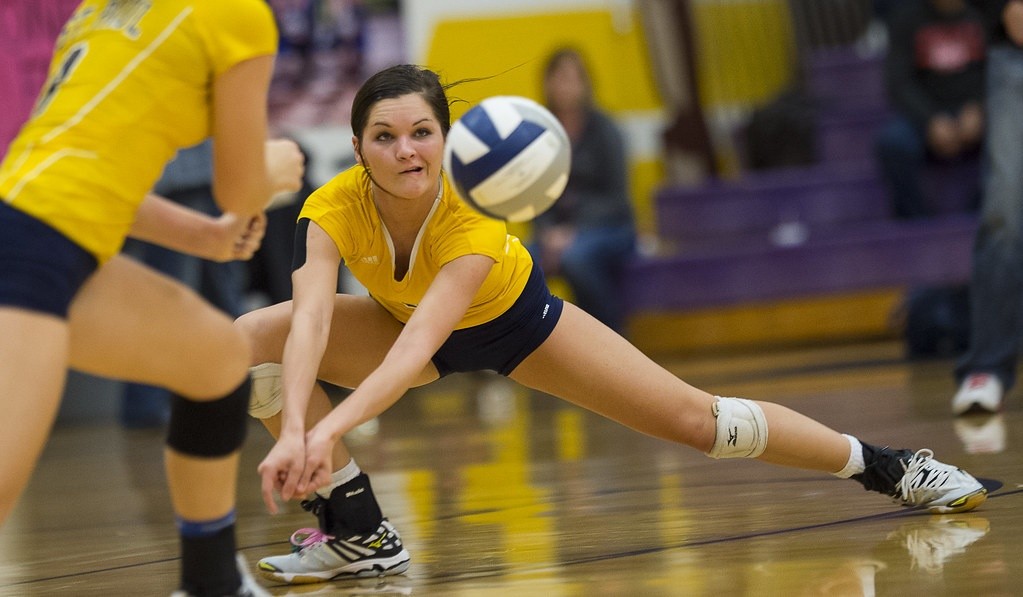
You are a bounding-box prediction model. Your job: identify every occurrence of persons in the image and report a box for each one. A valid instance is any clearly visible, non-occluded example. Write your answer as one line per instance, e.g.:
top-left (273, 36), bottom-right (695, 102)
top-left (0, 1), bottom-right (304, 597)
top-left (230, 61), bottom-right (985, 584)
top-left (875, 0), bottom-right (1023, 415)
top-left (526, 44), bottom-right (637, 332)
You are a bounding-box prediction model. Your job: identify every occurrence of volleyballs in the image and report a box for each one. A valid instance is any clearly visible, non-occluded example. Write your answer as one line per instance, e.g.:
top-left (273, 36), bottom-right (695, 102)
top-left (443, 95), bottom-right (571, 223)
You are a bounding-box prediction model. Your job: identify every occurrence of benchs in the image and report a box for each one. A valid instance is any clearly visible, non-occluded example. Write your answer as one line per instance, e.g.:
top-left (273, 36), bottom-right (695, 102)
top-left (630, 46), bottom-right (979, 356)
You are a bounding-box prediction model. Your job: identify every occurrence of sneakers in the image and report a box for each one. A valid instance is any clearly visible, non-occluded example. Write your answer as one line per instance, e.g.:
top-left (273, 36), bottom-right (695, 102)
top-left (253, 516), bottom-right (410, 582)
top-left (277, 575), bottom-right (412, 597)
top-left (952, 371), bottom-right (1006, 415)
top-left (888, 447), bottom-right (988, 514)
top-left (954, 413), bottom-right (1006, 454)
top-left (886, 511), bottom-right (989, 574)
